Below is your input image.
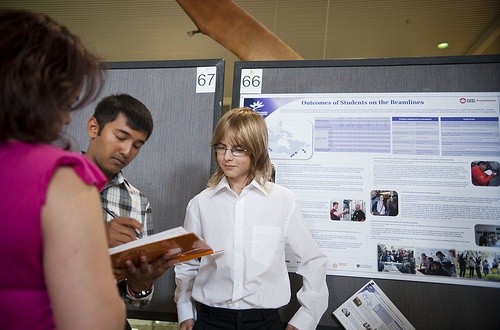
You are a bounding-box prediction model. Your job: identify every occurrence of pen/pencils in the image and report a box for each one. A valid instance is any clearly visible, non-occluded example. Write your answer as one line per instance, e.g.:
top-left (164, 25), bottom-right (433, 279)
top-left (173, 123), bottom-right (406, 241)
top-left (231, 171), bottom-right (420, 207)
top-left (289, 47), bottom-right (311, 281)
top-left (103, 206), bottom-right (143, 240)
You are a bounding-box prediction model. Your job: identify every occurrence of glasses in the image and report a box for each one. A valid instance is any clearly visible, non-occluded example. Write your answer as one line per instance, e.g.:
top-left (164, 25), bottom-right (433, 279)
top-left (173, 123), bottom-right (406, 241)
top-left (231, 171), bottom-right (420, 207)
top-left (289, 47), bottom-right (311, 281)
top-left (209, 143), bottom-right (249, 156)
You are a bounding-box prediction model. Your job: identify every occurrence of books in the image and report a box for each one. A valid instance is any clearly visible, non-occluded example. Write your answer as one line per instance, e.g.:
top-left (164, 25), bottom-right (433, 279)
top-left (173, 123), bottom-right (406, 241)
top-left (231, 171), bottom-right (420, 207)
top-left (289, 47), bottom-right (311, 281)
top-left (107, 226), bottom-right (225, 280)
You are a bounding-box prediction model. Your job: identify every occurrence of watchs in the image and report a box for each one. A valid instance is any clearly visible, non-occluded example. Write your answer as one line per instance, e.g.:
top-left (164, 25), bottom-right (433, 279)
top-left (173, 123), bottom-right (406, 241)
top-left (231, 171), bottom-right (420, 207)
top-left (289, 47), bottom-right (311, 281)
top-left (129, 284), bottom-right (153, 299)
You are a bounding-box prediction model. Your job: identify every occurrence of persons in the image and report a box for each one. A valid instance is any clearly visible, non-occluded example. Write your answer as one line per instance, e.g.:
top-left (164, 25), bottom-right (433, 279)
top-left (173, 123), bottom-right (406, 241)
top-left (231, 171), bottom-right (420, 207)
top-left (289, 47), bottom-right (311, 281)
top-left (377, 246), bottom-right (500, 282)
top-left (0, 8), bottom-right (130, 329)
top-left (173, 106), bottom-right (329, 329)
top-left (471, 161), bottom-right (497, 187)
top-left (79, 93), bottom-right (155, 303)
top-left (371, 190), bottom-right (398, 217)
top-left (331, 200), bottom-right (366, 222)
